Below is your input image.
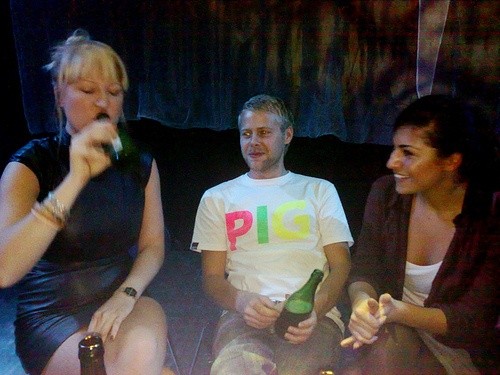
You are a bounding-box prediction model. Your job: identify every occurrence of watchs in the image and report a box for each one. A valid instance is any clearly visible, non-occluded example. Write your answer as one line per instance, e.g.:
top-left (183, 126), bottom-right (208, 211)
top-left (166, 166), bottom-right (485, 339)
top-left (114, 287), bottom-right (140, 301)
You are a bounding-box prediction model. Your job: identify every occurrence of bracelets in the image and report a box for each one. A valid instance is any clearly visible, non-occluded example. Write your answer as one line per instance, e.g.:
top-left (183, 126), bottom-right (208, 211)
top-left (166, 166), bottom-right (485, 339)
top-left (29, 191), bottom-right (70, 232)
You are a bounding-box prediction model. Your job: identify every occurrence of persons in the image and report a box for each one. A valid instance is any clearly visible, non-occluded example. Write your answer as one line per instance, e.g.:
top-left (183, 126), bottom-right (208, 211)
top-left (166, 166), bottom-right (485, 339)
top-left (0, 34), bottom-right (169, 375)
top-left (188, 95), bottom-right (354, 375)
top-left (339, 94), bottom-right (500, 375)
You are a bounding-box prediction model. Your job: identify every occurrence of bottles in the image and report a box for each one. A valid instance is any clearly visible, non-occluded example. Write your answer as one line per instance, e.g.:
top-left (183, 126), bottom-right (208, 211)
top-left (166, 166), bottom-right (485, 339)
top-left (96, 113), bottom-right (151, 193)
top-left (78, 335), bottom-right (107, 375)
top-left (274, 269), bottom-right (324, 341)
